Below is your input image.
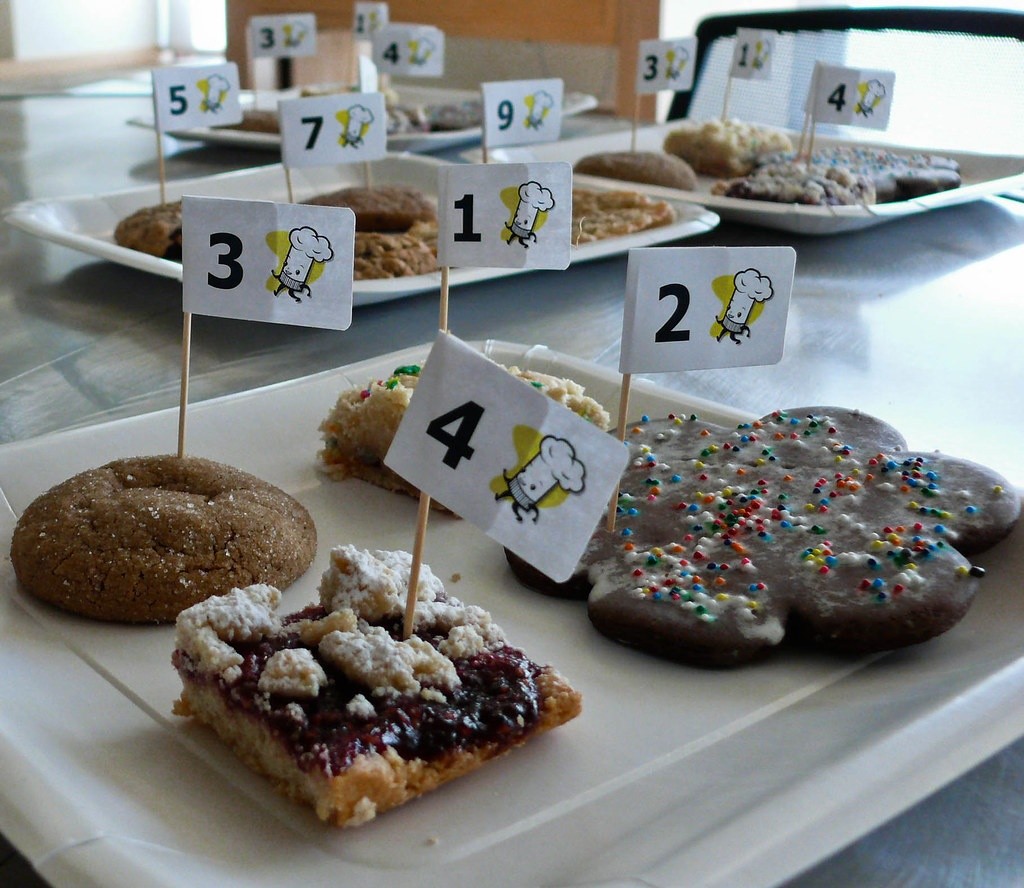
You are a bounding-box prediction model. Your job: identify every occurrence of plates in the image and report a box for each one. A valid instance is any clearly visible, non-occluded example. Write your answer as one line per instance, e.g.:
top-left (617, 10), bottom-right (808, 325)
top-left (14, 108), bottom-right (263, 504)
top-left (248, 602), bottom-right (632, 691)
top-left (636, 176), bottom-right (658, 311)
top-left (2, 152), bottom-right (722, 304)
top-left (464, 95), bottom-right (1023, 235)
top-left (126, 57), bottom-right (599, 151)
top-left (2, 335), bottom-right (1023, 888)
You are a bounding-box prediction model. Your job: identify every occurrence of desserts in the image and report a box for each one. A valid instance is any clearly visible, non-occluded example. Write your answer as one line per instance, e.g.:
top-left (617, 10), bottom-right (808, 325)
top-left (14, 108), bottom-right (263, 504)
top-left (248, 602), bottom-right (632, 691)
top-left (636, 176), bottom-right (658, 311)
top-left (319, 358), bottom-right (611, 485)
top-left (509, 404), bottom-right (1023, 661)
top-left (171, 541), bottom-right (584, 824)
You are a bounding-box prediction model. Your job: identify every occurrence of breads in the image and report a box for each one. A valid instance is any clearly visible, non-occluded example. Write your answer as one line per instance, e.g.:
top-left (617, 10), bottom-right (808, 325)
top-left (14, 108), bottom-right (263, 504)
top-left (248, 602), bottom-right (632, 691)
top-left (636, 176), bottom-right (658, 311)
top-left (10, 454), bottom-right (315, 628)
